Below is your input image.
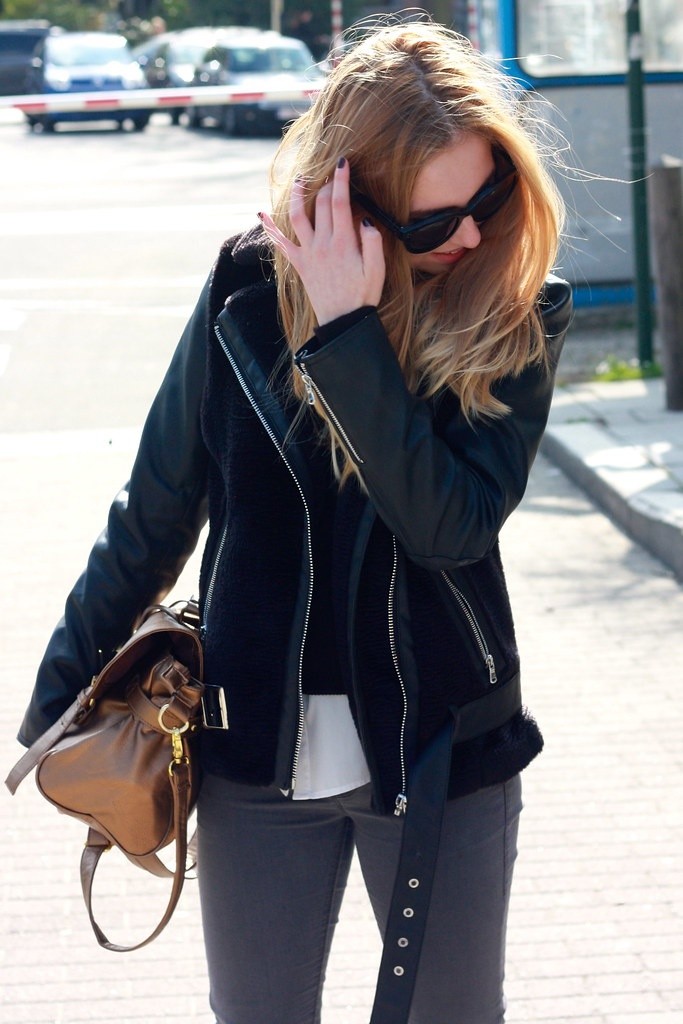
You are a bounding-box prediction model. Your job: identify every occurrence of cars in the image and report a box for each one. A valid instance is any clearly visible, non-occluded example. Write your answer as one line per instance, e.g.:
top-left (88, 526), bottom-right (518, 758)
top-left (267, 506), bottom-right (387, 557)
top-left (189, 41), bottom-right (322, 136)
top-left (162, 25), bottom-right (259, 127)
top-left (134, 32), bottom-right (174, 88)
top-left (0, 19), bottom-right (52, 97)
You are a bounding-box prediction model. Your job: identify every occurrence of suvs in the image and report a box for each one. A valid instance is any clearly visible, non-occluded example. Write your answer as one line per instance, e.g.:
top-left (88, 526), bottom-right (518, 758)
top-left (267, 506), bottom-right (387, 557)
top-left (21, 31), bottom-right (154, 132)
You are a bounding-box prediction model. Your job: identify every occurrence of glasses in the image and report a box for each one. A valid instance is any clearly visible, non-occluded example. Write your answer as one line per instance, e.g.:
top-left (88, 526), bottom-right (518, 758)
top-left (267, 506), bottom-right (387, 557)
top-left (350, 140), bottom-right (519, 255)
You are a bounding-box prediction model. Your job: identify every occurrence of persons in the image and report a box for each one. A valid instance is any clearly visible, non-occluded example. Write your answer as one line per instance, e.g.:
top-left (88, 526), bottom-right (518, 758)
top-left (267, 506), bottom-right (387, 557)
top-left (18, 21), bottom-right (574, 1024)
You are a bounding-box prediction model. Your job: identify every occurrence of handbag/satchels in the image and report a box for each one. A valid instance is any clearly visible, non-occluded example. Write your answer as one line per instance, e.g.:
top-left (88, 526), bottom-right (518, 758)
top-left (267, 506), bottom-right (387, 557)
top-left (4, 594), bottom-right (206, 953)
